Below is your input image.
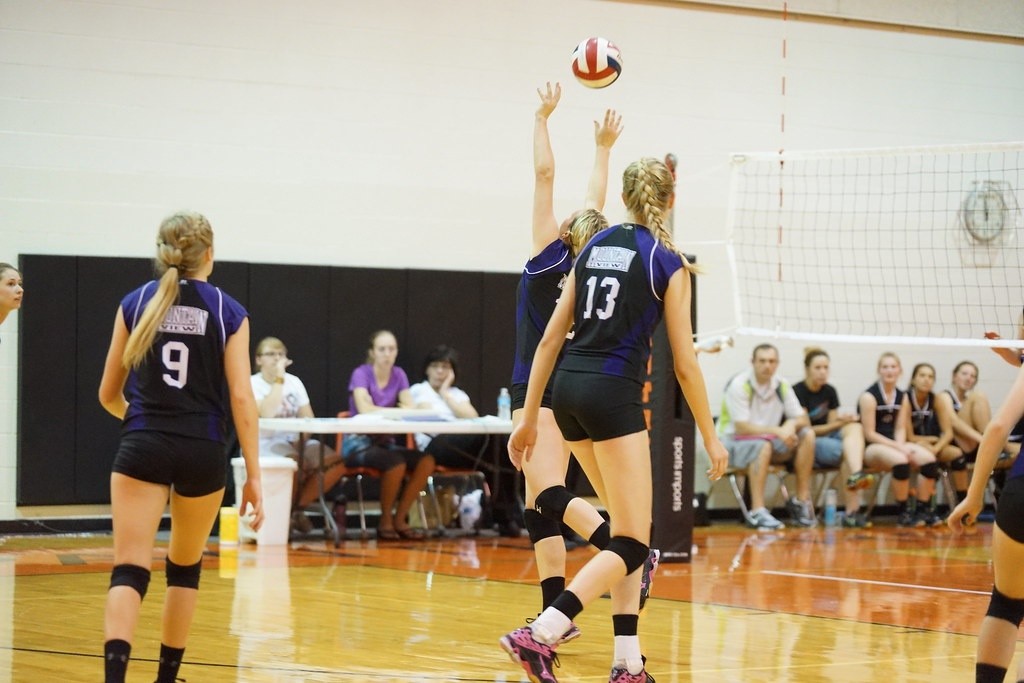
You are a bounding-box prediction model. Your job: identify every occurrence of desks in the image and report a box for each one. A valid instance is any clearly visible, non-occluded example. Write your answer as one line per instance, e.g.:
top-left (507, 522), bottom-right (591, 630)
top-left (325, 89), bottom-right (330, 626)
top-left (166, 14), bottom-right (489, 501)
top-left (255, 416), bottom-right (521, 549)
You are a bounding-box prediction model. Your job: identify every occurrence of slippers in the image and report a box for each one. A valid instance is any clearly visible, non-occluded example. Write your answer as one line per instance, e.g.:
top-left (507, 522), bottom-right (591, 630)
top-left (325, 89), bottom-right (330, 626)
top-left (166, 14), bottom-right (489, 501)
top-left (377, 528), bottom-right (400, 541)
top-left (399, 528), bottom-right (424, 540)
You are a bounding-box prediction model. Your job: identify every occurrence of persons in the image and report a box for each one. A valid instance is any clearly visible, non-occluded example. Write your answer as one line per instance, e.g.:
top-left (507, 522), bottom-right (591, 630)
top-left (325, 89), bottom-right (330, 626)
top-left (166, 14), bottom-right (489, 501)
top-left (251, 337), bottom-right (344, 532)
top-left (341, 331), bottom-right (527, 541)
top-left (499, 159), bottom-right (730, 683)
top-left (99, 214), bottom-right (266, 683)
top-left (716, 344), bottom-right (1020, 532)
top-left (984, 308), bottom-right (1024, 367)
top-left (513, 82), bottom-right (660, 643)
top-left (948, 364), bottom-right (1024, 683)
top-left (0, 262), bottom-right (24, 326)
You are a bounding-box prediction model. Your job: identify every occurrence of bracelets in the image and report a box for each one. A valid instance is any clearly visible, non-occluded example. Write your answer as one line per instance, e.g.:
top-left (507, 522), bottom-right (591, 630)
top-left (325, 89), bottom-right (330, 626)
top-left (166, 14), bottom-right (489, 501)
top-left (276, 378), bottom-right (284, 383)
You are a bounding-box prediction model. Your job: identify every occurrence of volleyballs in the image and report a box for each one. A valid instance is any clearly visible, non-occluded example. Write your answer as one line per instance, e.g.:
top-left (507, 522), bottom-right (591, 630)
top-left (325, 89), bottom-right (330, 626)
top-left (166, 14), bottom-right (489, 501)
top-left (569, 35), bottom-right (623, 89)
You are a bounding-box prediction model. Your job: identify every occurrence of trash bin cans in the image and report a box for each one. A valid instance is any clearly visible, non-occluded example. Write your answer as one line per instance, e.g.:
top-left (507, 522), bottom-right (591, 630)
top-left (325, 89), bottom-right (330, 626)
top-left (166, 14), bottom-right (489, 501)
top-left (232, 457), bottom-right (299, 545)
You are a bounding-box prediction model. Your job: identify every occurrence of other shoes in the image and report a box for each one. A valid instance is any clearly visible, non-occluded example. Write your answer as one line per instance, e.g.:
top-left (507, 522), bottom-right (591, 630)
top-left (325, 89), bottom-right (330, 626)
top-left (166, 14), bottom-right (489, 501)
top-left (292, 510), bottom-right (313, 532)
top-left (567, 534), bottom-right (589, 546)
top-left (500, 521), bottom-right (529, 536)
top-left (958, 516), bottom-right (977, 534)
top-left (565, 539), bottom-right (575, 550)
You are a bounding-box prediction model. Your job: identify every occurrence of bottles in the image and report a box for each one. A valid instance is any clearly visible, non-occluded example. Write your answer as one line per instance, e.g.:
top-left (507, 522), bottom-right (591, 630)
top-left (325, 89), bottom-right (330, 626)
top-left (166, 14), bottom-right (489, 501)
top-left (497, 388), bottom-right (511, 422)
top-left (823, 489), bottom-right (837, 526)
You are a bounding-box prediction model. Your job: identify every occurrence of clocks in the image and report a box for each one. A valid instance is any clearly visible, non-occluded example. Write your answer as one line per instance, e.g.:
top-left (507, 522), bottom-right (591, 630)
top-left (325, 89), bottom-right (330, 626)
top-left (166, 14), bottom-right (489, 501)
top-left (965, 187), bottom-right (1007, 240)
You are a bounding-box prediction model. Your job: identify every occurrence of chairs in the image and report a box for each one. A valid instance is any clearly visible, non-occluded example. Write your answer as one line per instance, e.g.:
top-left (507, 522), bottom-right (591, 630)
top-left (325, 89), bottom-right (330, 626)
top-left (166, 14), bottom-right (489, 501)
top-left (288, 413), bottom-right (493, 544)
top-left (698, 418), bottom-right (1001, 526)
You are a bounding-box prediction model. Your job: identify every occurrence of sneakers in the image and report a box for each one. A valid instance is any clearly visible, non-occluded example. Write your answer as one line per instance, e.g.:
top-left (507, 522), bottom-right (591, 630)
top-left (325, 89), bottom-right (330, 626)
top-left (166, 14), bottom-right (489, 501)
top-left (787, 496), bottom-right (818, 528)
top-left (846, 473), bottom-right (874, 491)
top-left (499, 627), bottom-right (561, 683)
top-left (919, 509), bottom-right (942, 528)
top-left (746, 506), bottom-right (785, 530)
top-left (638, 549), bottom-right (661, 614)
top-left (897, 510), bottom-right (926, 528)
top-left (842, 510), bottom-right (873, 529)
top-left (526, 613), bottom-right (581, 644)
top-left (609, 655), bottom-right (656, 683)
